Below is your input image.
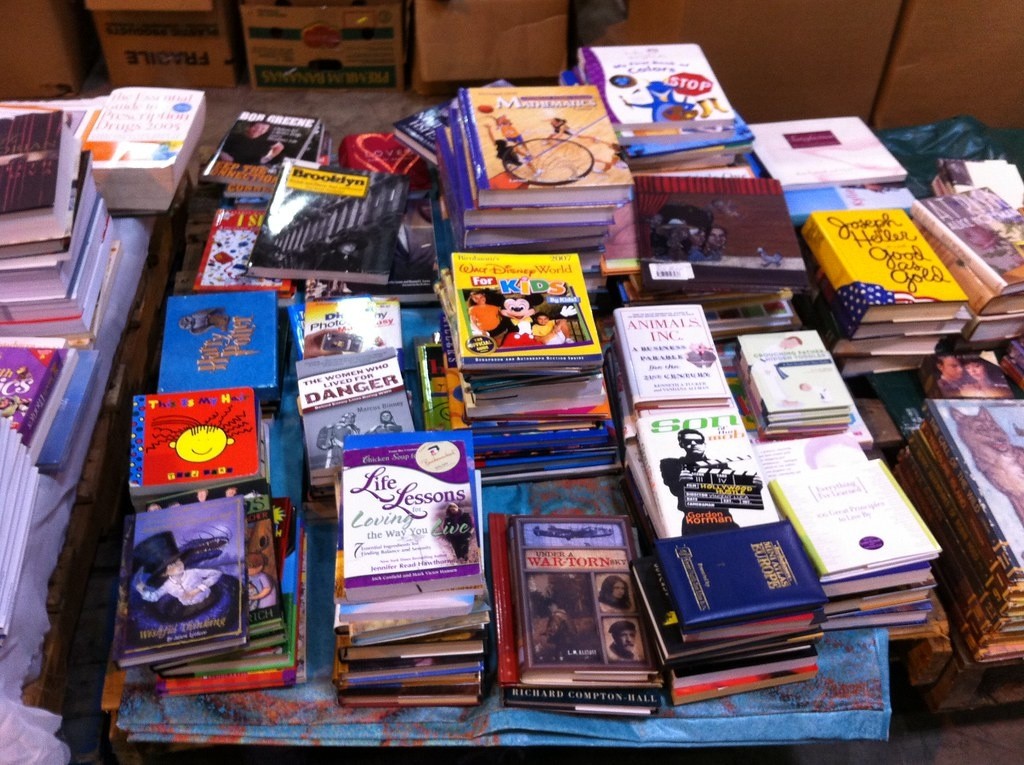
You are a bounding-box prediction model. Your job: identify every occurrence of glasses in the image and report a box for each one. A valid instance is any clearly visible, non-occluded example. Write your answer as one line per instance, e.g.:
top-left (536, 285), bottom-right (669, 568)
top-left (684, 437), bottom-right (704, 445)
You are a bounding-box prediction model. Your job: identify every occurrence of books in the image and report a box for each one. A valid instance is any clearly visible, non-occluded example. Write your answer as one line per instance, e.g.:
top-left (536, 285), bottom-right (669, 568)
top-left (1, 41), bottom-right (1023, 696)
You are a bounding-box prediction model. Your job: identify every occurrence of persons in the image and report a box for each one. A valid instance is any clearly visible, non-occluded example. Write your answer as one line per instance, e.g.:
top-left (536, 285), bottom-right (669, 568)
top-left (599, 576), bottom-right (633, 613)
top-left (960, 355), bottom-right (1012, 398)
top-left (222, 122), bottom-right (283, 165)
top-left (660, 429), bottom-right (740, 533)
top-left (607, 621), bottom-right (639, 661)
top-left (444, 503), bottom-right (473, 559)
top-left (934, 353), bottom-right (975, 397)
top-left (365, 410), bottom-right (404, 433)
top-left (327, 413), bottom-right (359, 467)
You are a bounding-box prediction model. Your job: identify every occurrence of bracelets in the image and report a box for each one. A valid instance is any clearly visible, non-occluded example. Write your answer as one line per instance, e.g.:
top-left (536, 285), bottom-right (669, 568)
top-left (266, 155), bottom-right (271, 161)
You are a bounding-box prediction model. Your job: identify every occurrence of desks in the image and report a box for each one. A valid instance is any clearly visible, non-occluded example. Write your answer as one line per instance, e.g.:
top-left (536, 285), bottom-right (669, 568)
top-left (1, 100), bottom-right (1024, 765)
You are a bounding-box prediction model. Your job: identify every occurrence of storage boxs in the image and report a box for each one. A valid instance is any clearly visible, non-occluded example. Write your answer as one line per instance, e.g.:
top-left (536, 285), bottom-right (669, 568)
top-left (0, 0), bottom-right (1024, 137)
